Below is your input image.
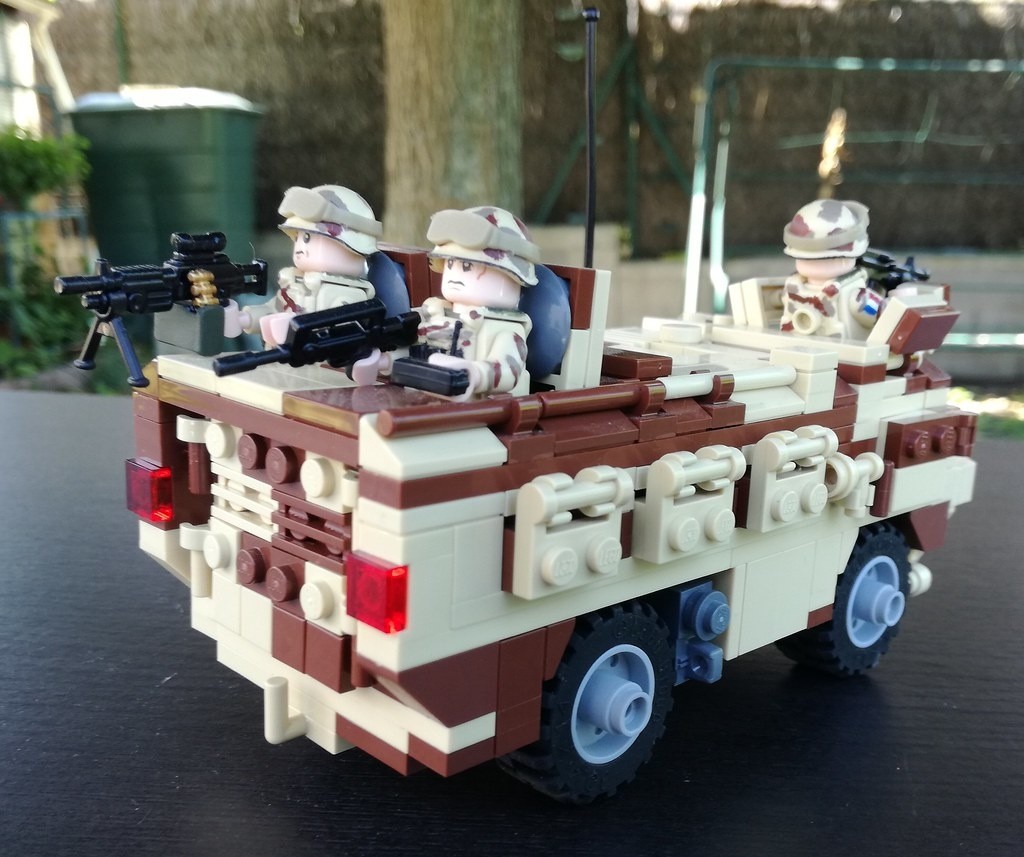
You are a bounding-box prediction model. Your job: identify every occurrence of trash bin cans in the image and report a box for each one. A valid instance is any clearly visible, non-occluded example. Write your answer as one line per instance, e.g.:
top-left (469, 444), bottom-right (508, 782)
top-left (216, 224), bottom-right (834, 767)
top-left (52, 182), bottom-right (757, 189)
top-left (60, 87), bottom-right (265, 347)
